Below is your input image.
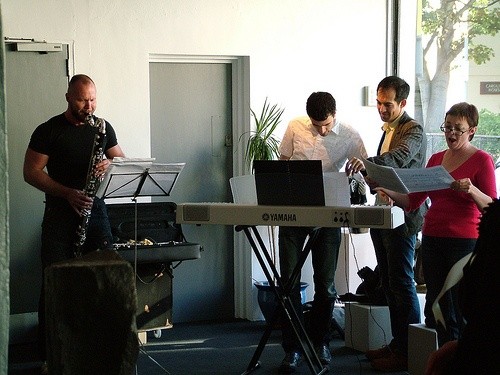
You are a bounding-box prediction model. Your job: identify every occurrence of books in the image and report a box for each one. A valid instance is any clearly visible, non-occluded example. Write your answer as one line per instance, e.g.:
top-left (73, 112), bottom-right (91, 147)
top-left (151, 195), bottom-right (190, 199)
top-left (360, 154), bottom-right (456, 196)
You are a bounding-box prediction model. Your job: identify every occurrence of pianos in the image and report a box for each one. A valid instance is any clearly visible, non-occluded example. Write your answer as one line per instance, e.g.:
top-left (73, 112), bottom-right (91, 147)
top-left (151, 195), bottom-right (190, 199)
top-left (176, 202), bottom-right (405, 375)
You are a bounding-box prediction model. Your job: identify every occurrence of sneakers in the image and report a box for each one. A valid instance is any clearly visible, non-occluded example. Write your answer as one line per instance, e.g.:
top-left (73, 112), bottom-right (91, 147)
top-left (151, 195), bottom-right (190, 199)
top-left (364, 346), bottom-right (409, 372)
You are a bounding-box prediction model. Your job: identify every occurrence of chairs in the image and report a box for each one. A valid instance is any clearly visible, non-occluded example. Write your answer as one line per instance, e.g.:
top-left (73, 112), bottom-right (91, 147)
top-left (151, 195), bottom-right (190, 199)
top-left (38, 259), bottom-right (140, 375)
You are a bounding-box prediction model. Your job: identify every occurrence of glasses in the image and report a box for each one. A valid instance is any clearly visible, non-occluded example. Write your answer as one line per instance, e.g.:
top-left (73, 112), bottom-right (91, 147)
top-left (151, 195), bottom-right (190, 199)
top-left (440, 120), bottom-right (471, 136)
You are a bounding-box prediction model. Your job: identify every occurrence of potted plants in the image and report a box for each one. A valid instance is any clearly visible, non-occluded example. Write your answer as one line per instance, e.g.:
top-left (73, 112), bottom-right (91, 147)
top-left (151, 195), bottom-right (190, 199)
top-left (237, 96), bottom-right (309, 327)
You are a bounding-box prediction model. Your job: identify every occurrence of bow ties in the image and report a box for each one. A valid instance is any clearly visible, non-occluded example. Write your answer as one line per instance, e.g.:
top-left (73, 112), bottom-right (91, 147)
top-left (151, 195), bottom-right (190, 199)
top-left (383, 123), bottom-right (391, 134)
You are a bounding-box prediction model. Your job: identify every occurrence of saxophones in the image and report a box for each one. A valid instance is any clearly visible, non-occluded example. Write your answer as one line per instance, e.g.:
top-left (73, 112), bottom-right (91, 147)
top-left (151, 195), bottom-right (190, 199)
top-left (73, 114), bottom-right (108, 259)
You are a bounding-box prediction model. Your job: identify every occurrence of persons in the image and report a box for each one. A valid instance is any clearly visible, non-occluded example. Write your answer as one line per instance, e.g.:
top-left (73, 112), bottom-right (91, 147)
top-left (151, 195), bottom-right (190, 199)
top-left (24, 74), bottom-right (125, 375)
top-left (279, 91), bottom-right (368, 369)
top-left (458, 198), bottom-right (500, 375)
top-left (345, 76), bottom-right (427, 372)
top-left (369, 102), bottom-right (498, 347)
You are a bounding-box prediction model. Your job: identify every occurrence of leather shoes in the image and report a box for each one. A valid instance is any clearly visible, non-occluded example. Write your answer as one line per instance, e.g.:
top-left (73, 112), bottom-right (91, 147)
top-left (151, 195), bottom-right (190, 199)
top-left (280, 352), bottom-right (301, 372)
top-left (314, 343), bottom-right (334, 365)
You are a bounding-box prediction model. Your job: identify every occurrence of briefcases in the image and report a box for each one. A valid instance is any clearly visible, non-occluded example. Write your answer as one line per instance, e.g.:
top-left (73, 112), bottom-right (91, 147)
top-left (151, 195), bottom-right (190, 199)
top-left (107, 201), bottom-right (204, 284)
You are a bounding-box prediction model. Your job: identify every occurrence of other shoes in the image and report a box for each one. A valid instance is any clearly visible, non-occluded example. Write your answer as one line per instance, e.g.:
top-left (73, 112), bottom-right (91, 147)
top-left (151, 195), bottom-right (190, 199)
top-left (40, 361), bottom-right (48, 375)
